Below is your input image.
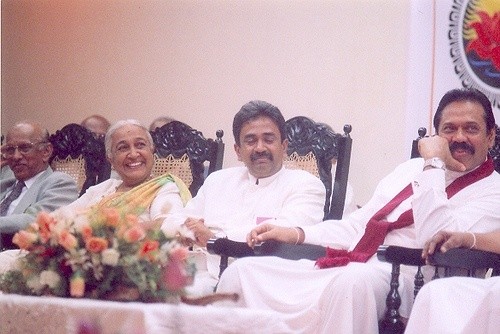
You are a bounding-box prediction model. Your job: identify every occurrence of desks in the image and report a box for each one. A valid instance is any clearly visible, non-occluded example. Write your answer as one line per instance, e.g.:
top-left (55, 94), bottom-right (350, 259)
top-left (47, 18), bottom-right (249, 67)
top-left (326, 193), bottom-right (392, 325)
top-left (0, 292), bottom-right (282, 334)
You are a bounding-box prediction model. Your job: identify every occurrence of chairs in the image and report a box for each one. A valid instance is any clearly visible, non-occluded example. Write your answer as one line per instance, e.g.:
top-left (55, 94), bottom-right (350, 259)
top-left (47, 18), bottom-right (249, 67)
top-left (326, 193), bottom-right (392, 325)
top-left (206, 116), bottom-right (353, 293)
top-left (48, 123), bottom-right (112, 199)
top-left (148, 121), bottom-right (225, 199)
top-left (251, 124), bottom-right (500, 334)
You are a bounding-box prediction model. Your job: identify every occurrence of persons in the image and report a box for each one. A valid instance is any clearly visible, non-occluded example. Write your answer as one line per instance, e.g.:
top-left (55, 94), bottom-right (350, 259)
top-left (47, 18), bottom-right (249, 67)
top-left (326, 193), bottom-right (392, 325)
top-left (161, 100), bottom-right (325, 298)
top-left (50, 120), bottom-right (192, 237)
top-left (215, 87), bottom-right (500, 334)
top-left (0, 121), bottom-right (76, 234)
top-left (403, 229), bottom-right (500, 334)
top-left (150, 117), bottom-right (173, 132)
top-left (82, 116), bottom-right (111, 136)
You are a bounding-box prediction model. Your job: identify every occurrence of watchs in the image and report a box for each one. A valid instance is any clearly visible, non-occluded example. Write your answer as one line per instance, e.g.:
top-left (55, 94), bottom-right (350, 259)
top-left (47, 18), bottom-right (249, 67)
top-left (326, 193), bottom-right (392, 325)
top-left (206, 233), bottom-right (227, 254)
top-left (422, 157), bottom-right (447, 171)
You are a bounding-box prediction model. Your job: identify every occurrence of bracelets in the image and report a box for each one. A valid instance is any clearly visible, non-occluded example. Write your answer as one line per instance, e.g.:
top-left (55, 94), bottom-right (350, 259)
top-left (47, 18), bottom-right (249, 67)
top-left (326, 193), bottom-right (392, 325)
top-left (294, 228), bottom-right (299, 245)
top-left (468, 231), bottom-right (476, 250)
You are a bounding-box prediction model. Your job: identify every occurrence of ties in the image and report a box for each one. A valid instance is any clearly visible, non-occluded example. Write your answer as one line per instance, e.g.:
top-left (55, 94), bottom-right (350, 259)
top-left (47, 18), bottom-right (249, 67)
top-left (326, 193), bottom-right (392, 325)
top-left (0, 181), bottom-right (25, 216)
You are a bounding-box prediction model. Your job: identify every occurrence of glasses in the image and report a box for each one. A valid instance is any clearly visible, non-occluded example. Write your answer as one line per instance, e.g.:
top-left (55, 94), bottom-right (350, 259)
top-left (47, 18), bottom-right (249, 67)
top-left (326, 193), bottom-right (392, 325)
top-left (1, 141), bottom-right (46, 157)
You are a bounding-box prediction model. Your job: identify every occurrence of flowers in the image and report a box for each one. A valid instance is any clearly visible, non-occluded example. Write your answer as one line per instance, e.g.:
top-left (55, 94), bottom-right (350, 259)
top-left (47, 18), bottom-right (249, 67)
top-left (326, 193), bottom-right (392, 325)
top-left (0, 209), bottom-right (192, 302)
